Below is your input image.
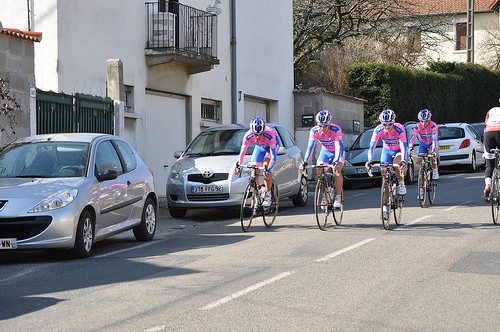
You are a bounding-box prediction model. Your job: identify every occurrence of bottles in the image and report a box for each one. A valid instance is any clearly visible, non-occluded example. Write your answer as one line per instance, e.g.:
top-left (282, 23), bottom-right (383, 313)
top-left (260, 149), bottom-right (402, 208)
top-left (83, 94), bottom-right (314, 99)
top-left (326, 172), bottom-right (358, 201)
top-left (330, 186), bottom-right (334, 198)
top-left (257, 185), bottom-right (261, 198)
top-left (393, 182), bottom-right (396, 195)
top-left (427, 170), bottom-right (430, 180)
top-left (261, 184), bottom-right (266, 198)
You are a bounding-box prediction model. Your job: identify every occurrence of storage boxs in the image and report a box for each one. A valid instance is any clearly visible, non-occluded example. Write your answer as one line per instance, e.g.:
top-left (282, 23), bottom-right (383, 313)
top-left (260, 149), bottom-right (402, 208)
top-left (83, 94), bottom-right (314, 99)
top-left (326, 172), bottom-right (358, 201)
top-left (151, 12), bottom-right (176, 47)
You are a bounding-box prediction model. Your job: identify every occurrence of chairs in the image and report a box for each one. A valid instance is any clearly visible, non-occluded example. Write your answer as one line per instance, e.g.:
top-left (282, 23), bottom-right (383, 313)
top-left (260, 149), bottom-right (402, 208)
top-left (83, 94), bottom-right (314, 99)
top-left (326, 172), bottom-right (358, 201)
top-left (28, 152), bottom-right (54, 175)
top-left (216, 132), bottom-right (239, 149)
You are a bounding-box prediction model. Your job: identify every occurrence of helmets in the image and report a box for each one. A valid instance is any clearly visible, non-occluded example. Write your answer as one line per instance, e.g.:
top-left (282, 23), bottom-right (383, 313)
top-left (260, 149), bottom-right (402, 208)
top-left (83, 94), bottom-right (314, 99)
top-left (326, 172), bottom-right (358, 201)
top-left (379, 109), bottom-right (396, 123)
top-left (315, 109), bottom-right (333, 124)
top-left (250, 117), bottom-right (265, 134)
top-left (417, 109), bottom-right (432, 121)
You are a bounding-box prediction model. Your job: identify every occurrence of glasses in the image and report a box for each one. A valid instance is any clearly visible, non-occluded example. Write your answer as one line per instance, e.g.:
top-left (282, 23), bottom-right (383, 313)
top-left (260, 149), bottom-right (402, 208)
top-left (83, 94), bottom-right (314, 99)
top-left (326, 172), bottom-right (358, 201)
top-left (382, 123), bottom-right (393, 126)
top-left (254, 133), bottom-right (263, 136)
top-left (420, 120), bottom-right (429, 123)
top-left (319, 124), bottom-right (329, 127)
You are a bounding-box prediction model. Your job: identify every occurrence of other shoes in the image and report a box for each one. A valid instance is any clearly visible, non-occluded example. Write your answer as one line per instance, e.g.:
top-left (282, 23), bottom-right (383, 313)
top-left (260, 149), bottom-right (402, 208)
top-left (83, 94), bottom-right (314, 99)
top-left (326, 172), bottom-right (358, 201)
top-left (432, 165), bottom-right (439, 181)
top-left (333, 194), bottom-right (342, 208)
top-left (398, 184), bottom-right (407, 195)
top-left (483, 184), bottom-right (492, 204)
top-left (380, 210), bottom-right (389, 220)
top-left (416, 188), bottom-right (424, 200)
top-left (261, 196), bottom-right (272, 207)
top-left (252, 203), bottom-right (260, 216)
top-left (320, 198), bottom-right (328, 210)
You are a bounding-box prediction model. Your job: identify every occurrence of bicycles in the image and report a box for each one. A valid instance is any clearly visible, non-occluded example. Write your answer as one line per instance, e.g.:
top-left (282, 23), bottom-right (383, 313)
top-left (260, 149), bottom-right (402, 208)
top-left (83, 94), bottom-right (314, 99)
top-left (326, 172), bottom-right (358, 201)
top-left (406, 151), bottom-right (438, 207)
top-left (303, 162), bottom-right (343, 231)
top-left (365, 163), bottom-right (403, 230)
top-left (485, 148), bottom-right (500, 227)
top-left (235, 161), bottom-right (279, 232)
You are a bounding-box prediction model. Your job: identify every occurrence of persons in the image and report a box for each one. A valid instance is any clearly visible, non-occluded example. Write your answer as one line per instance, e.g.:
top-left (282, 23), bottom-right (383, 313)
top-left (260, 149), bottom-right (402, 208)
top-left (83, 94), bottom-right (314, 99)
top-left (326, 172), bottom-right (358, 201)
top-left (234, 116), bottom-right (280, 207)
top-left (409, 109), bottom-right (439, 199)
top-left (365, 109), bottom-right (410, 220)
top-left (299, 110), bottom-right (345, 209)
top-left (482, 100), bottom-right (500, 204)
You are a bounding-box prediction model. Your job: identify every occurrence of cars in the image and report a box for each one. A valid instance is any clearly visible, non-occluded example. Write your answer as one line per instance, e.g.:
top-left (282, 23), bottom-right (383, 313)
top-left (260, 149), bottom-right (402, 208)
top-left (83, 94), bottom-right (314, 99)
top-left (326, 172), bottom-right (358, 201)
top-left (343, 119), bottom-right (423, 189)
top-left (434, 122), bottom-right (489, 174)
top-left (166, 123), bottom-right (309, 221)
top-left (0, 131), bottom-right (159, 258)
